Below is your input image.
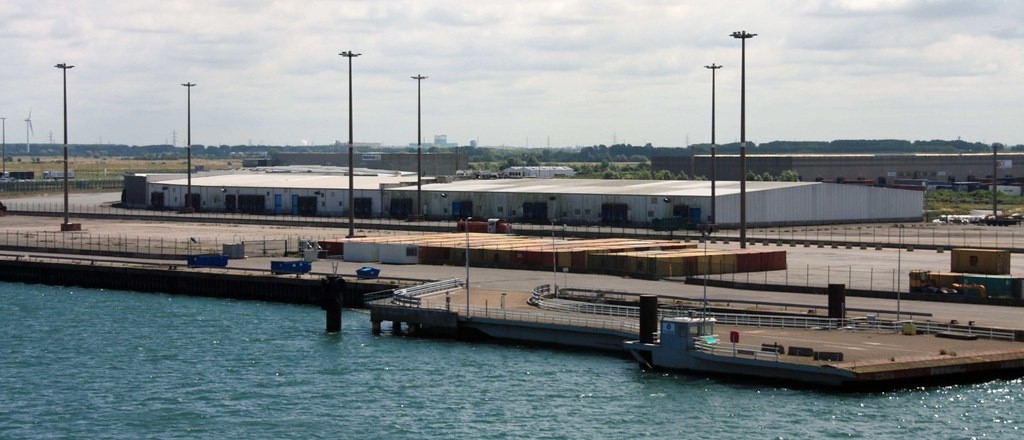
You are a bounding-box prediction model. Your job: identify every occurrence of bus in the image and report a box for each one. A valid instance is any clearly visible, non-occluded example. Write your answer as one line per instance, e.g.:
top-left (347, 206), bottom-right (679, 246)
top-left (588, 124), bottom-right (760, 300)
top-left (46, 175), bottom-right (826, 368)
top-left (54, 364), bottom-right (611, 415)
top-left (926, 181), bottom-right (1024, 195)
top-left (820, 176), bottom-right (876, 187)
top-left (887, 178), bottom-right (929, 192)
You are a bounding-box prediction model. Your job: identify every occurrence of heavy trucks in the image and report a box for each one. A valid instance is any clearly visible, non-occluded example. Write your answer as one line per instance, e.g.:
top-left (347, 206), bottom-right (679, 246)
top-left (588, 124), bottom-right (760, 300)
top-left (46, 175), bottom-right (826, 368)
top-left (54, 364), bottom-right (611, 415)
top-left (5, 171), bottom-right (35, 180)
top-left (44, 170), bottom-right (75, 181)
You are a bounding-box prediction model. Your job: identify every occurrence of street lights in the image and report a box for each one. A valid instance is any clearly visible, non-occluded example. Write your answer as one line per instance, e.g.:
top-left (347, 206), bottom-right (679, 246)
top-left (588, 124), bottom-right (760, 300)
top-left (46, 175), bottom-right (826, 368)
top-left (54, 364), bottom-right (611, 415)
top-left (180, 81), bottom-right (197, 209)
top-left (412, 73), bottom-right (429, 221)
top-left (55, 62), bottom-right (74, 225)
top-left (338, 49), bottom-right (362, 238)
top-left (704, 61), bottom-right (724, 236)
top-left (727, 31), bottom-right (758, 248)
